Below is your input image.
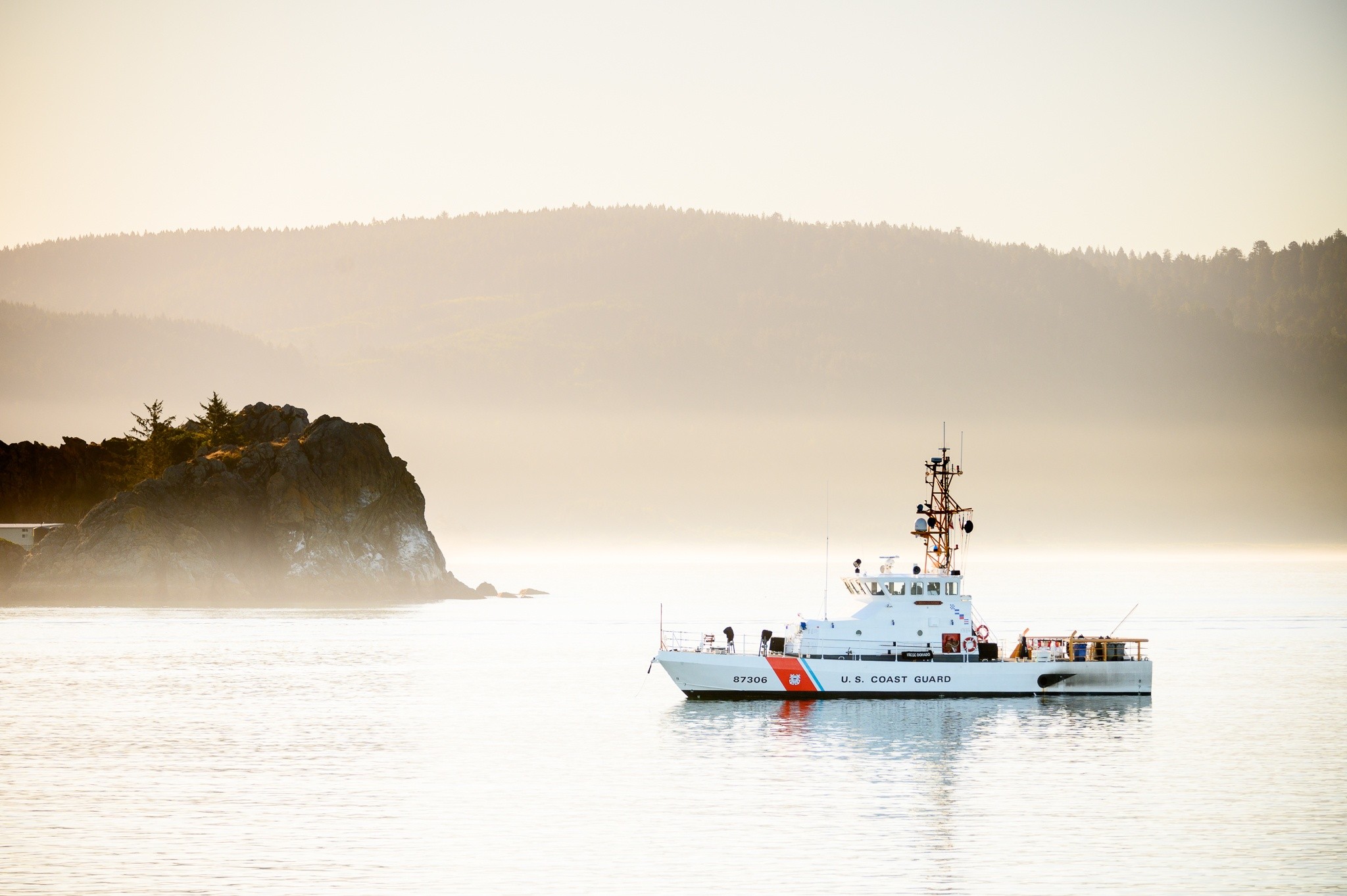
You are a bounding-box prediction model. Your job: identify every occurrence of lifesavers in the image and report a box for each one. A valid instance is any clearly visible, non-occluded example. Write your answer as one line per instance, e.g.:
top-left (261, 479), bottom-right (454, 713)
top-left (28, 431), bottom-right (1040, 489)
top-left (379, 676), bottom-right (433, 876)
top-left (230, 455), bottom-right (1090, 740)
top-left (977, 625), bottom-right (989, 639)
top-left (963, 637), bottom-right (976, 652)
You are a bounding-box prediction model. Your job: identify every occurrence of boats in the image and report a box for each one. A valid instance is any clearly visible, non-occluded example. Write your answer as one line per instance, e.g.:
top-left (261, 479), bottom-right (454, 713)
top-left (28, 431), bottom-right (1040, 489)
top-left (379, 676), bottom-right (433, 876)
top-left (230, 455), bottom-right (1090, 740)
top-left (646, 421), bottom-right (1153, 702)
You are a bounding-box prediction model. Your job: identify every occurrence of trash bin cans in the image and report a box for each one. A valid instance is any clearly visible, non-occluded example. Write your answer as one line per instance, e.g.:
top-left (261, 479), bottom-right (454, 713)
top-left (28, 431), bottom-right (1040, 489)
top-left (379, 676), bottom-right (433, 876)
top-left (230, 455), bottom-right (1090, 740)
top-left (1073, 643), bottom-right (1087, 662)
top-left (1114, 642), bottom-right (1126, 661)
top-left (1101, 642), bottom-right (1118, 661)
